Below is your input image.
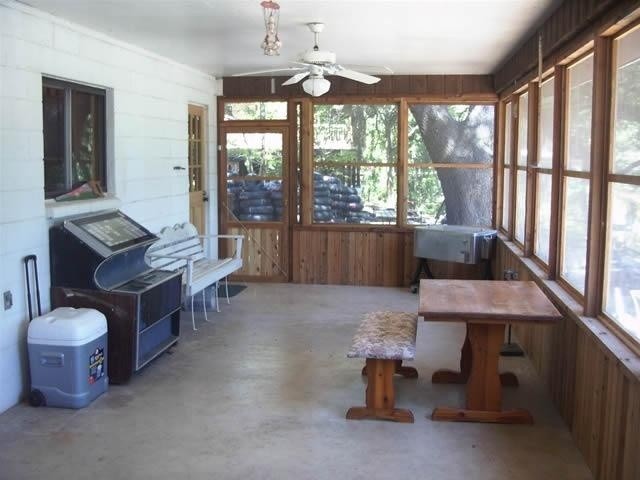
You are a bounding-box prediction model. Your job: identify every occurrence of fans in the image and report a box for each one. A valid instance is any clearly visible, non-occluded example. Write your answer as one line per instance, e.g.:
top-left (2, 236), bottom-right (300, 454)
top-left (230, 22), bottom-right (394, 97)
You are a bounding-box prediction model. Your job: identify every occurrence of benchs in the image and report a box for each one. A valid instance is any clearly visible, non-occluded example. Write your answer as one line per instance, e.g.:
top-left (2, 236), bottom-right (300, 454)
top-left (144, 222), bottom-right (245, 331)
top-left (346, 310), bottom-right (417, 423)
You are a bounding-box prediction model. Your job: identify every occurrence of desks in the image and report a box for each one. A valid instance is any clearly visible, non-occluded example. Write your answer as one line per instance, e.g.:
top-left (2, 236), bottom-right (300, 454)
top-left (418, 279), bottom-right (563, 425)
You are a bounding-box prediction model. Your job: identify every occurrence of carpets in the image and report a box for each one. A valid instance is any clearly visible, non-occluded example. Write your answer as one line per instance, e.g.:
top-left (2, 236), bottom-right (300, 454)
top-left (217, 285), bottom-right (248, 297)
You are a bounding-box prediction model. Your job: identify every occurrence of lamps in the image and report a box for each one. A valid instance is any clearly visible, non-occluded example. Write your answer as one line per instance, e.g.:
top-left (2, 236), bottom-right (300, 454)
top-left (260, 0), bottom-right (282, 56)
top-left (301, 76), bottom-right (332, 97)
